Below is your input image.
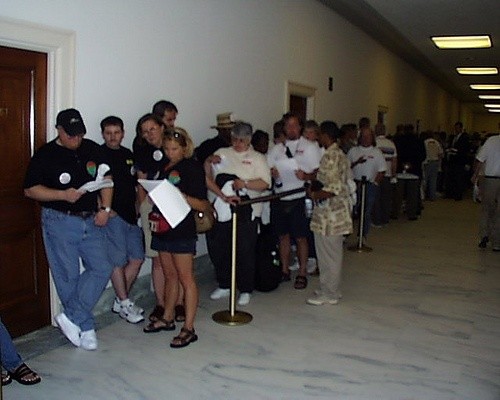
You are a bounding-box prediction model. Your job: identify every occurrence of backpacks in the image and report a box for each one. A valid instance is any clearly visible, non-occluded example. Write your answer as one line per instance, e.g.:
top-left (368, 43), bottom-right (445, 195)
top-left (256, 233), bottom-right (280, 292)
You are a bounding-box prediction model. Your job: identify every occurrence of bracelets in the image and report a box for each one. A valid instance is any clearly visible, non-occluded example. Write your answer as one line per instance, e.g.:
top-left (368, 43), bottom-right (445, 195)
top-left (244, 179), bottom-right (248, 188)
top-left (310, 191), bottom-right (315, 197)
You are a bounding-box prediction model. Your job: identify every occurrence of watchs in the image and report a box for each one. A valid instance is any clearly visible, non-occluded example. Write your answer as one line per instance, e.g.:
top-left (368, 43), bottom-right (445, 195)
top-left (100, 206), bottom-right (111, 213)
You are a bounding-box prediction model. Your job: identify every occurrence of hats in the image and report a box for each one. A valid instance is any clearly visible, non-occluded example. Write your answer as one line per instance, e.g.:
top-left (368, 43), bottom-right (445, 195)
top-left (209, 111), bottom-right (239, 129)
top-left (54, 108), bottom-right (86, 137)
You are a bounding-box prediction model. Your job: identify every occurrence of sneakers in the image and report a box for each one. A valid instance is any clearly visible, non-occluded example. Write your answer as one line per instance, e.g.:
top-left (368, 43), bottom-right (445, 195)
top-left (288, 260), bottom-right (300, 271)
top-left (314, 289), bottom-right (343, 299)
top-left (112, 297), bottom-right (144, 315)
top-left (291, 244), bottom-right (297, 251)
top-left (307, 293), bottom-right (337, 305)
top-left (306, 257), bottom-right (317, 274)
top-left (55, 312), bottom-right (81, 348)
top-left (119, 299), bottom-right (145, 324)
top-left (80, 328), bottom-right (98, 350)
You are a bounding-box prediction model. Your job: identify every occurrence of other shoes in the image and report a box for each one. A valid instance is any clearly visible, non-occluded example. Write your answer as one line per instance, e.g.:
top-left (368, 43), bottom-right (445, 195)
top-left (238, 292), bottom-right (249, 305)
top-left (209, 287), bottom-right (230, 299)
top-left (493, 248), bottom-right (500, 252)
top-left (478, 236), bottom-right (488, 249)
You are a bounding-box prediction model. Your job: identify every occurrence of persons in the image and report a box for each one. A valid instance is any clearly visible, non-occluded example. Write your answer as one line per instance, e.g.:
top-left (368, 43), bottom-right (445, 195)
top-left (136, 113), bottom-right (187, 322)
top-left (472, 124), bottom-right (500, 252)
top-left (424, 122), bottom-right (482, 202)
top-left (97, 116), bottom-right (146, 323)
top-left (346, 127), bottom-right (386, 249)
top-left (370, 123), bottom-right (426, 229)
top-left (306, 120), bottom-right (356, 305)
top-left (193, 112), bottom-right (273, 305)
top-left (0, 320), bottom-right (41, 385)
top-left (24, 109), bottom-right (114, 351)
top-left (267, 113), bottom-right (321, 289)
top-left (143, 127), bottom-right (210, 348)
top-left (251, 112), bottom-right (358, 154)
top-left (133, 100), bottom-right (178, 156)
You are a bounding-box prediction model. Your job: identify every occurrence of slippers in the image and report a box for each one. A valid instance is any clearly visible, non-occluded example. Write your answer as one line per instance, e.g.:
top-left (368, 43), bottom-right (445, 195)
top-left (175, 304), bottom-right (186, 322)
top-left (149, 305), bottom-right (164, 322)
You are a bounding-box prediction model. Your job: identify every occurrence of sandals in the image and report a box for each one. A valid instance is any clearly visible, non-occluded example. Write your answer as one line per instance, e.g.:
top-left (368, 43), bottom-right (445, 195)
top-left (10, 363), bottom-right (41, 385)
top-left (170, 327), bottom-right (198, 348)
top-left (294, 275), bottom-right (307, 289)
top-left (1, 372), bottom-right (12, 386)
top-left (280, 269), bottom-right (291, 282)
top-left (143, 317), bottom-right (176, 333)
